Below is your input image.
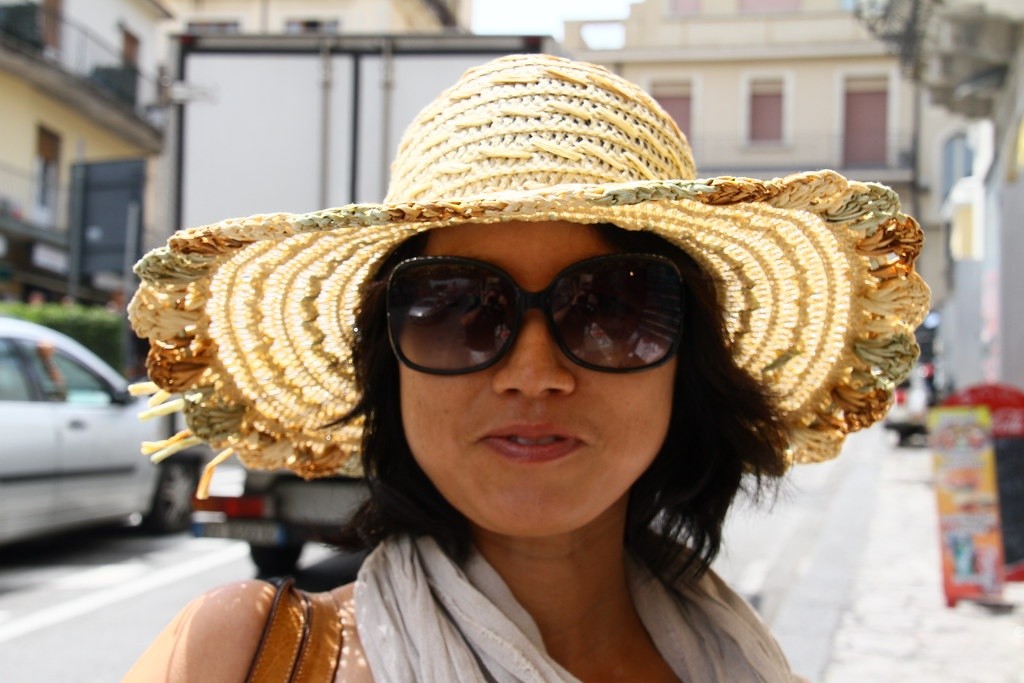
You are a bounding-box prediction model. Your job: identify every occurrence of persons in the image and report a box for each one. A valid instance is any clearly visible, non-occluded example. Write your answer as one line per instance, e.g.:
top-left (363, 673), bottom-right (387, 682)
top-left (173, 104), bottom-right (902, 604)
top-left (116, 55), bottom-right (932, 683)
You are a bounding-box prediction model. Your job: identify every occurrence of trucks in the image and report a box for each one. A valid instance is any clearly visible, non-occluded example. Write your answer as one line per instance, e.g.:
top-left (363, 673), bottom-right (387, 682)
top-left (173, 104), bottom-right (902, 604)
top-left (155, 18), bottom-right (571, 578)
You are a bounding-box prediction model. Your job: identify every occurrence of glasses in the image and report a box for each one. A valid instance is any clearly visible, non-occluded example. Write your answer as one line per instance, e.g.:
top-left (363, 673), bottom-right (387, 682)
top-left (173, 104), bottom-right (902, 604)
top-left (355, 254), bottom-right (690, 376)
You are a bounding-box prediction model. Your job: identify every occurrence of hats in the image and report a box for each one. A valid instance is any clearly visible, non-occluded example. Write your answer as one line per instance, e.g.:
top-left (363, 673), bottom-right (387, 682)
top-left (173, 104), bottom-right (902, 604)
top-left (126, 53), bottom-right (930, 480)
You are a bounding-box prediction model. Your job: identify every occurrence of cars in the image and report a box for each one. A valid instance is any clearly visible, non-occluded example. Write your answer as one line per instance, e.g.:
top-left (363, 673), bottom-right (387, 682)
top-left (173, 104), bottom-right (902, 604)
top-left (0, 318), bottom-right (202, 547)
top-left (881, 315), bottom-right (941, 451)
